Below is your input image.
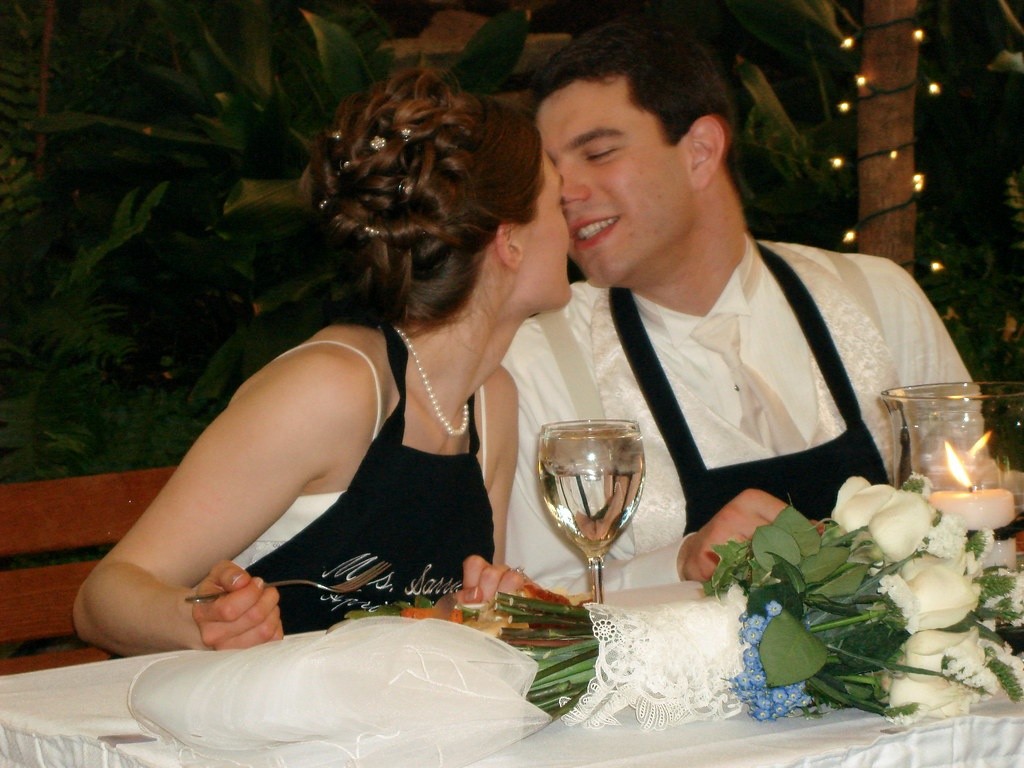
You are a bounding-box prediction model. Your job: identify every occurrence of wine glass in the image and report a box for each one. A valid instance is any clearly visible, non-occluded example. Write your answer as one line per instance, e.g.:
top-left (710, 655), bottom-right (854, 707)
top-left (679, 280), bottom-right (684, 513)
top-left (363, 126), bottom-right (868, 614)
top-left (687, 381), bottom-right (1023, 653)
top-left (539, 419), bottom-right (646, 605)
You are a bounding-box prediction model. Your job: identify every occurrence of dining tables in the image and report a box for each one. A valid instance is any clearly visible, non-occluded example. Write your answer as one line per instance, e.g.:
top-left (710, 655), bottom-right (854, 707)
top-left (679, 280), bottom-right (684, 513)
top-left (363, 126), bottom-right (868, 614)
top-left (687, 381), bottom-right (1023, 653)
top-left (1, 622), bottom-right (1024, 768)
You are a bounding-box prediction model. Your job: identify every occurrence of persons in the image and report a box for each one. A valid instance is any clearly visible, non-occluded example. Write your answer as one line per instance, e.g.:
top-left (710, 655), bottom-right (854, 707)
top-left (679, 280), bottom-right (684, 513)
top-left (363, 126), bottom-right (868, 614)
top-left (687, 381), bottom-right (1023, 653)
top-left (500, 19), bottom-right (981, 595)
top-left (72, 67), bottom-right (572, 657)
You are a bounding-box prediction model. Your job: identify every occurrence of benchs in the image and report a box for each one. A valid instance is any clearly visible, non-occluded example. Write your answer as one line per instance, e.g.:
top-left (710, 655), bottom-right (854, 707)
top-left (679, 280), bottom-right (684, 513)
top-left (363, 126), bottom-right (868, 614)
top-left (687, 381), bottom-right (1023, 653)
top-left (0, 466), bottom-right (178, 673)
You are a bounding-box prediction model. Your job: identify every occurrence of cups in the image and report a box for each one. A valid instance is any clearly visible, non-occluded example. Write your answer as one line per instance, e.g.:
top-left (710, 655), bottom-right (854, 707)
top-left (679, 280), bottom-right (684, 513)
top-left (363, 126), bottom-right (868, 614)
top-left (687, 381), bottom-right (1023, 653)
top-left (881, 383), bottom-right (1024, 569)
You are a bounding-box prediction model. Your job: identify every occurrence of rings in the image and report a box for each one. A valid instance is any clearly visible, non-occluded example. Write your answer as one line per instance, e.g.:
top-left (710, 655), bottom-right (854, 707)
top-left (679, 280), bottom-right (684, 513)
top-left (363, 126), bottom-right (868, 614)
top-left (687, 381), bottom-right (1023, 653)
top-left (509, 567), bottom-right (527, 579)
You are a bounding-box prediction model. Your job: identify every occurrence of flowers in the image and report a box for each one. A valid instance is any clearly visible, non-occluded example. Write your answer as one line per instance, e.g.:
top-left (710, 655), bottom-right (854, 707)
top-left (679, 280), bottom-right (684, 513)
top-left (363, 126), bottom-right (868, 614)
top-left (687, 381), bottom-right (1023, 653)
top-left (488, 477), bottom-right (1024, 721)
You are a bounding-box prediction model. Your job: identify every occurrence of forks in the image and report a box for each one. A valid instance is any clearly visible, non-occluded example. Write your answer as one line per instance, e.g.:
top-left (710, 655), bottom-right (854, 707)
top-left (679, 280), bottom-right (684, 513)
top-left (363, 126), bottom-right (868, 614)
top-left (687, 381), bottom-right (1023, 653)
top-left (185, 561), bottom-right (392, 604)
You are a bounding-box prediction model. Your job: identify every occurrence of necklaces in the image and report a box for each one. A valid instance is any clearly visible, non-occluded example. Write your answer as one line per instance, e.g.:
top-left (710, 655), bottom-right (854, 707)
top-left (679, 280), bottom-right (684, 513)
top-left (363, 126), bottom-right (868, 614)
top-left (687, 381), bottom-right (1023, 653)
top-left (394, 325), bottom-right (469, 436)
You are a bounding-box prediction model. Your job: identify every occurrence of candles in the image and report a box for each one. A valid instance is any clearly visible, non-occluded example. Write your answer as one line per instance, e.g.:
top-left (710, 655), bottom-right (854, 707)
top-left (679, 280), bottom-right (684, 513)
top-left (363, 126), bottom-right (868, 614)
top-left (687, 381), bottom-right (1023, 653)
top-left (930, 442), bottom-right (1019, 575)
